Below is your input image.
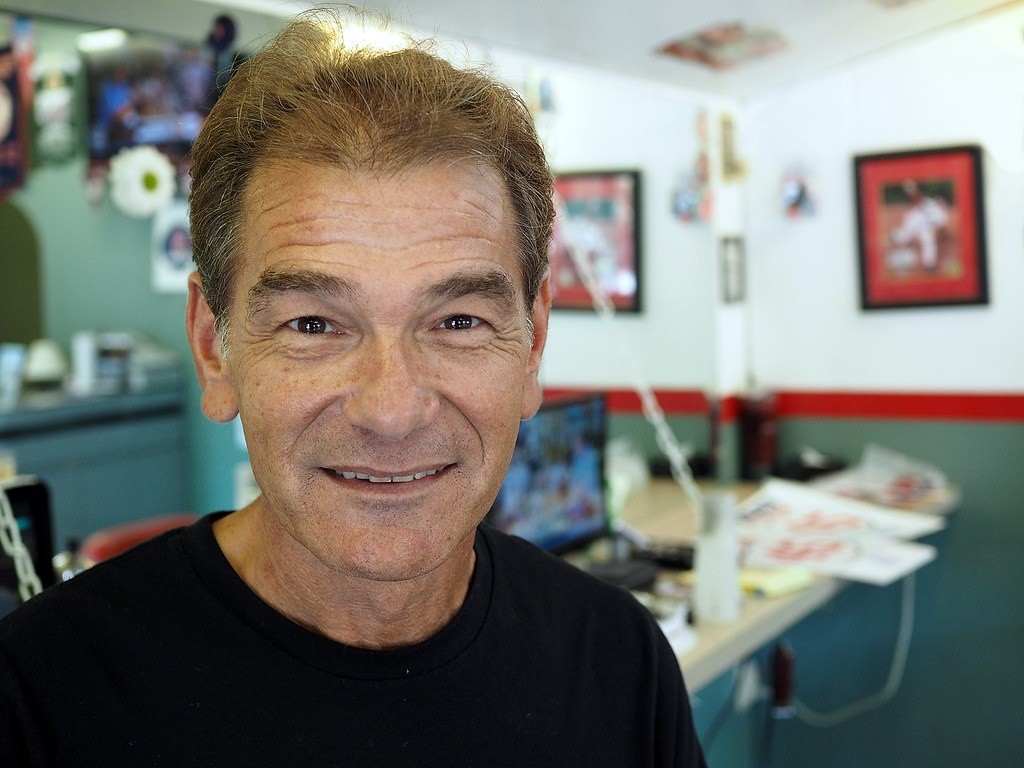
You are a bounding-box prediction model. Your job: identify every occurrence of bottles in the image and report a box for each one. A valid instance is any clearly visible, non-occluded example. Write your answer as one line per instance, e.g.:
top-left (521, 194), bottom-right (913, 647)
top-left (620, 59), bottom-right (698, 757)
top-left (691, 494), bottom-right (744, 629)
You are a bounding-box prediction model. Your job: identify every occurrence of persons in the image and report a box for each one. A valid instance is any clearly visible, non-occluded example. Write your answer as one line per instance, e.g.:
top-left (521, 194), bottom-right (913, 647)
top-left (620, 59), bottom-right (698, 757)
top-left (0, 22), bottom-right (708, 768)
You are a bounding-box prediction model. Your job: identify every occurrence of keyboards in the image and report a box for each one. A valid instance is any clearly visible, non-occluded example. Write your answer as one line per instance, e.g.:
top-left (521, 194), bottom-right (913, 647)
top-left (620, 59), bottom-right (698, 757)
top-left (586, 554), bottom-right (659, 591)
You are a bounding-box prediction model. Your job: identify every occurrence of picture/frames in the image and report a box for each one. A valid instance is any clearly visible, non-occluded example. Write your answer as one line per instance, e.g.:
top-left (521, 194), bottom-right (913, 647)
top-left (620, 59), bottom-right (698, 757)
top-left (544, 168), bottom-right (642, 311)
top-left (852, 145), bottom-right (987, 311)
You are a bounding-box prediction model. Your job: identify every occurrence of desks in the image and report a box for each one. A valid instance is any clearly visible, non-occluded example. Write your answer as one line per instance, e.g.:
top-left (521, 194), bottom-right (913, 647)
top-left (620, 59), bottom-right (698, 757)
top-left (559, 473), bottom-right (959, 681)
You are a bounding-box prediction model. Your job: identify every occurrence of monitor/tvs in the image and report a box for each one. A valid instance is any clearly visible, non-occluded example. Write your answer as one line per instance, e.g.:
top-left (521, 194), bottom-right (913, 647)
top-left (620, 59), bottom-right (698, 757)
top-left (480, 391), bottom-right (608, 558)
